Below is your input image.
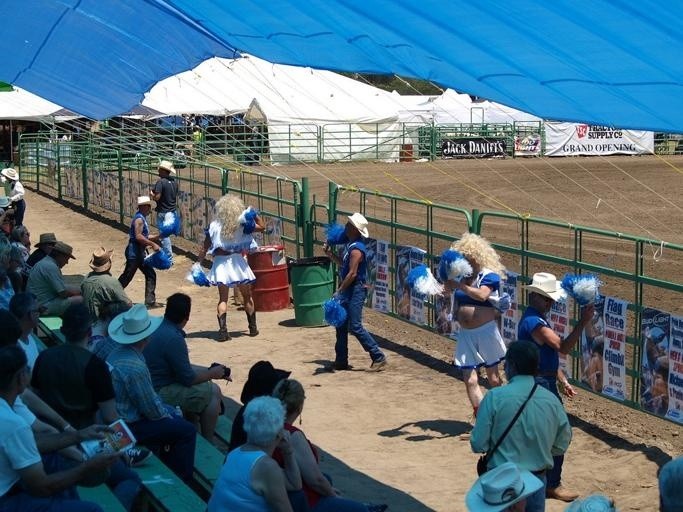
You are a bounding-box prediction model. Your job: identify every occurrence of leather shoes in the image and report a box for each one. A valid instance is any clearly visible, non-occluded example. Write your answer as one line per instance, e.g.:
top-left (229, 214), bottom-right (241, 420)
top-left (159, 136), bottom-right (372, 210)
top-left (327, 360), bottom-right (352, 370)
top-left (547, 485), bottom-right (578, 505)
top-left (365, 359), bottom-right (390, 373)
top-left (141, 299), bottom-right (166, 311)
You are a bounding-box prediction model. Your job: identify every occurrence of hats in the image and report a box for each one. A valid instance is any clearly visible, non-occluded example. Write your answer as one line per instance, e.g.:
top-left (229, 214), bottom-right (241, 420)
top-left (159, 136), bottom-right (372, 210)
top-left (521, 271), bottom-right (568, 302)
top-left (0, 167), bottom-right (20, 182)
top-left (1, 197), bottom-right (12, 208)
top-left (87, 246), bottom-right (115, 272)
top-left (136, 196), bottom-right (157, 208)
top-left (108, 302), bottom-right (164, 345)
top-left (52, 240), bottom-right (76, 260)
top-left (157, 160), bottom-right (177, 174)
top-left (33, 232), bottom-right (62, 246)
top-left (240, 360), bottom-right (292, 402)
top-left (347, 212), bottom-right (369, 238)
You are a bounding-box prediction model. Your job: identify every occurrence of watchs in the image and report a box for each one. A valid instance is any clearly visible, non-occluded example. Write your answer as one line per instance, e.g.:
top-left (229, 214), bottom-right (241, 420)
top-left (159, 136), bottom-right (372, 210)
top-left (79, 448), bottom-right (90, 464)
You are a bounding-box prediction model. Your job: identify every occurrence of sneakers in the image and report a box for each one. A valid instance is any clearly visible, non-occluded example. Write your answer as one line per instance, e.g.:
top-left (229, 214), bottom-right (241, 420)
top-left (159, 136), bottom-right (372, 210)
top-left (126, 444), bottom-right (152, 466)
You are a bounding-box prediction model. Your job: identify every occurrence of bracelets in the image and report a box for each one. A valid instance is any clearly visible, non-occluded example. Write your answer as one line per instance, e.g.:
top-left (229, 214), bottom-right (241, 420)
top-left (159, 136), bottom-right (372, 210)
top-left (63, 423), bottom-right (73, 434)
top-left (281, 449), bottom-right (295, 456)
top-left (198, 249), bottom-right (208, 252)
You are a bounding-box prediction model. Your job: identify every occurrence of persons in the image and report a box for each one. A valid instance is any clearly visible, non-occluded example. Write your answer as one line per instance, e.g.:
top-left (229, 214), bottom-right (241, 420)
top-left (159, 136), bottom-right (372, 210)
top-left (8, 292), bottom-right (42, 380)
top-left (143, 292), bottom-right (233, 453)
top-left (657, 455), bottom-right (683, 512)
top-left (0, 195), bottom-right (17, 235)
top-left (19, 232), bottom-right (59, 288)
top-left (195, 193), bottom-right (267, 343)
top-left (142, 159), bottom-right (181, 271)
top-left (467, 338), bottom-right (575, 510)
top-left (119, 194), bottom-right (165, 309)
top-left (1, 167), bottom-right (27, 225)
top-left (30, 302), bottom-right (123, 427)
top-left (1, 340), bottom-right (139, 512)
top-left (321, 211), bottom-right (388, 373)
top-left (24, 239), bottom-right (84, 316)
top-left (565, 493), bottom-right (619, 512)
top-left (0, 310), bottom-right (154, 466)
top-left (517, 267), bottom-right (604, 498)
top-left (403, 231), bottom-right (513, 416)
top-left (203, 393), bottom-right (308, 512)
top-left (10, 225), bottom-right (33, 278)
top-left (223, 360), bottom-right (293, 468)
top-left (94, 301), bottom-right (185, 439)
top-left (272, 378), bottom-right (390, 512)
top-left (0, 235), bottom-right (15, 310)
top-left (79, 245), bottom-right (134, 318)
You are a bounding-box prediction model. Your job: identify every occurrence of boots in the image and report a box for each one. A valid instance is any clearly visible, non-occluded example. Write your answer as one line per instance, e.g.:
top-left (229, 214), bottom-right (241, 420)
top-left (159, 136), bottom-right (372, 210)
top-left (217, 312), bottom-right (232, 341)
top-left (245, 309), bottom-right (259, 337)
